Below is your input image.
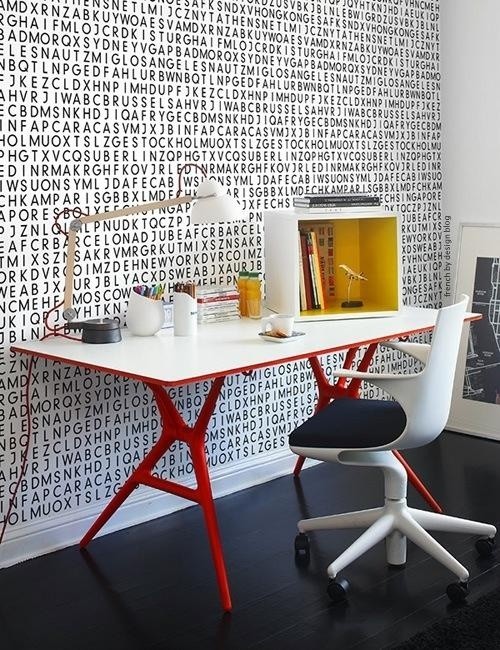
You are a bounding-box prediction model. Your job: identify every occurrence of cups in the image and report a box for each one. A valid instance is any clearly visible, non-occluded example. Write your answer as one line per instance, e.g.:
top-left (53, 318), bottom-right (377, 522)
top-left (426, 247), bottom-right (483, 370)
top-left (126, 288), bottom-right (165, 337)
top-left (261, 314), bottom-right (294, 335)
top-left (173, 292), bottom-right (197, 336)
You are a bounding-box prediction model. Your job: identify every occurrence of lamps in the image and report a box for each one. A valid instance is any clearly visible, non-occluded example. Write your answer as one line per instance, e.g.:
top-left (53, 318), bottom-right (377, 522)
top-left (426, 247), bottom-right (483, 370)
top-left (64, 164), bottom-right (245, 344)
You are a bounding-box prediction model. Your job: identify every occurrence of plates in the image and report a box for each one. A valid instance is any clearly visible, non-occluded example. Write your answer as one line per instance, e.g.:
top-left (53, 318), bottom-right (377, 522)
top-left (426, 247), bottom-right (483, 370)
top-left (258, 330), bottom-right (305, 343)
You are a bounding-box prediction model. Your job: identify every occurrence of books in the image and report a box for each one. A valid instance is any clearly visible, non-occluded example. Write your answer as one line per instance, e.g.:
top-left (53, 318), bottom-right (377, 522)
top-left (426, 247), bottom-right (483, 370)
top-left (292, 192), bottom-right (383, 215)
top-left (190, 283), bottom-right (241, 325)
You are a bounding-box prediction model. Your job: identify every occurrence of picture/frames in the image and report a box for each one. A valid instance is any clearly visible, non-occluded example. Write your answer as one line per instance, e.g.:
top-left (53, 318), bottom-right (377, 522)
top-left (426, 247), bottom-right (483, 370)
top-left (442, 222), bottom-right (498, 441)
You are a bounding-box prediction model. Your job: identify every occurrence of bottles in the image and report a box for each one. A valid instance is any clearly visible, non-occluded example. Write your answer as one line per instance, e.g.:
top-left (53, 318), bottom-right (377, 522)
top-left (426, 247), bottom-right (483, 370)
top-left (238, 272), bottom-right (262, 318)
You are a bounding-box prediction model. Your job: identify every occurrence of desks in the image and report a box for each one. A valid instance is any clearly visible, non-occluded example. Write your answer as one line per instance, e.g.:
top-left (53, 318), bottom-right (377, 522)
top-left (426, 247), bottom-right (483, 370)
top-left (8, 306), bottom-right (482, 613)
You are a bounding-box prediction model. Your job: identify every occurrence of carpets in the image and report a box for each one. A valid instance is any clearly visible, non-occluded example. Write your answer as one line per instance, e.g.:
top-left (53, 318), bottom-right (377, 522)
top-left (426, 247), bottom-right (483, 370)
top-left (380, 587), bottom-right (500, 649)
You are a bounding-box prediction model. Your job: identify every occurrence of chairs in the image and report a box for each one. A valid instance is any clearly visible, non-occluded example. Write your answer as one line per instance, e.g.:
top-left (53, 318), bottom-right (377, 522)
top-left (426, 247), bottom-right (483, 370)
top-left (287, 295), bottom-right (497, 607)
